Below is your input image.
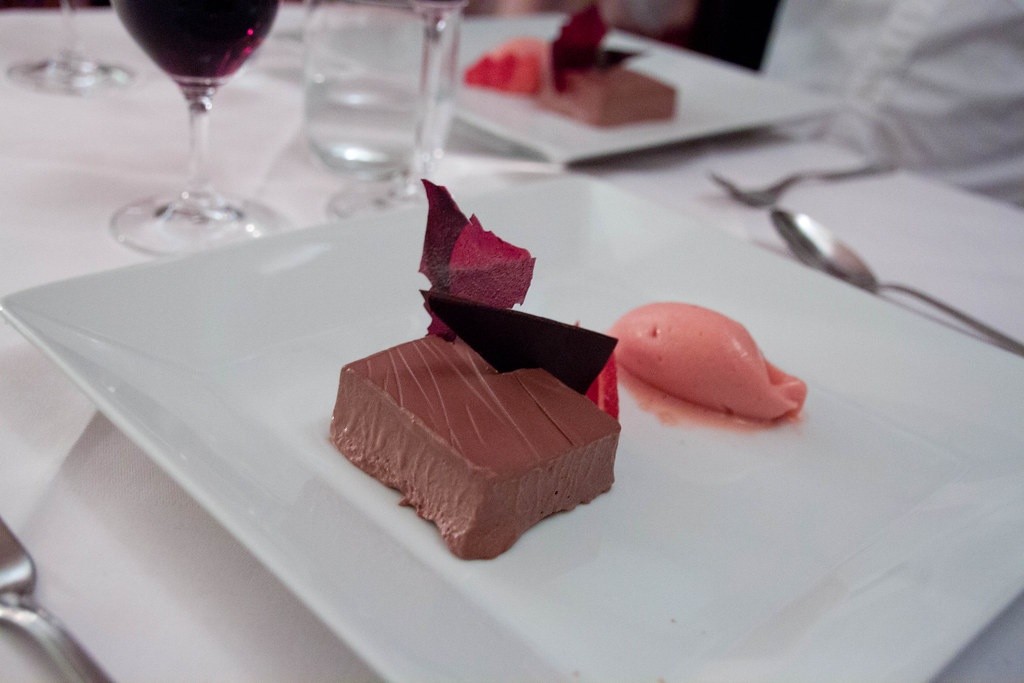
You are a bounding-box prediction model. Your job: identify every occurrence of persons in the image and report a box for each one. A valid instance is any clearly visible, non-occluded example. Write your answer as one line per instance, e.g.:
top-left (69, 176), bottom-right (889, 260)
top-left (666, 0), bottom-right (1024, 209)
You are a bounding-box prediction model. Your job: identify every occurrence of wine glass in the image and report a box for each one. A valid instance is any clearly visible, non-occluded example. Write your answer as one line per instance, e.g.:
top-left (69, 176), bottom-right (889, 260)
top-left (5, 0), bottom-right (136, 100)
top-left (108, 0), bottom-right (296, 253)
top-left (319, 1), bottom-right (469, 214)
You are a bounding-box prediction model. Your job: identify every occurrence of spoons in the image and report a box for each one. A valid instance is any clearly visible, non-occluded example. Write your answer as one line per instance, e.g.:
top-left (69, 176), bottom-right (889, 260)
top-left (768, 205), bottom-right (1024, 357)
top-left (0, 516), bottom-right (117, 682)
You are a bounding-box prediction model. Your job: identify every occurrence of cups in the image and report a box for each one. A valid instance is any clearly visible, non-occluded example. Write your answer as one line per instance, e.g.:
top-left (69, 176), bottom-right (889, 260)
top-left (300, 1), bottom-right (426, 168)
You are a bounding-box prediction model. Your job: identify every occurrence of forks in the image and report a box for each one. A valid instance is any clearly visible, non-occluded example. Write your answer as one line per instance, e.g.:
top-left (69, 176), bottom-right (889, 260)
top-left (706, 160), bottom-right (898, 206)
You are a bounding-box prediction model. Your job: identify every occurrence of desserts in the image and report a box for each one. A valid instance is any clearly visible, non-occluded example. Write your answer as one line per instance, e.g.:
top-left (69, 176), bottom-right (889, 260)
top-left (461, 38), bottom-right (545, 92)
top-left (533, 0), bottom-right (675, 127)
top-left (607, 299), bottom-right (805, 422)
top-left (326, 177), bottom-right (622, 562)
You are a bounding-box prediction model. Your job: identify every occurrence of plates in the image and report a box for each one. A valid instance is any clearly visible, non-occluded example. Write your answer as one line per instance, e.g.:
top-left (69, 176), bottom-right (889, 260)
top-left (310, 12), bottom-right (838, 160)
top-left (0, 172), bottom-right (1024, 681)
